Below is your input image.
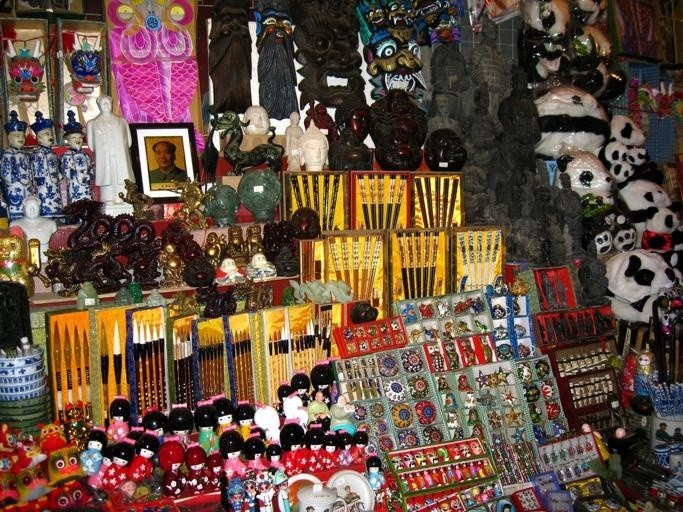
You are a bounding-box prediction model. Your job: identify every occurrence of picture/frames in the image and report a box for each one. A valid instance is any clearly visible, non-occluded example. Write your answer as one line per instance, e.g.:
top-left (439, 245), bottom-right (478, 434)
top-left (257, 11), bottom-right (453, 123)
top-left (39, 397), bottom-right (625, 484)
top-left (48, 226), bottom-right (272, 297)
top-left (127, 121), bottom-right (203, 204)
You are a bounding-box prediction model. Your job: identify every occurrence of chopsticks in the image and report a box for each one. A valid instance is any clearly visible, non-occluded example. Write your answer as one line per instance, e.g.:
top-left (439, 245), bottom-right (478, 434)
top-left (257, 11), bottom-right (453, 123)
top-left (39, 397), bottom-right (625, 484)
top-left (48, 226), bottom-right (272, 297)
top-left (290, 173), bottom-right (504, 308)
top-left (645, 380), bottom-right (682, 420)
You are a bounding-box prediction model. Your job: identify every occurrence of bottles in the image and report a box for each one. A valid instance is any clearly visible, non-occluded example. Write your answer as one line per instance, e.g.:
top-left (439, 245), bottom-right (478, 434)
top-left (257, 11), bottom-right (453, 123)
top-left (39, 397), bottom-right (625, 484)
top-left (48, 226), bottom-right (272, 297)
top-left (654, 442), bottom-right (681, 468)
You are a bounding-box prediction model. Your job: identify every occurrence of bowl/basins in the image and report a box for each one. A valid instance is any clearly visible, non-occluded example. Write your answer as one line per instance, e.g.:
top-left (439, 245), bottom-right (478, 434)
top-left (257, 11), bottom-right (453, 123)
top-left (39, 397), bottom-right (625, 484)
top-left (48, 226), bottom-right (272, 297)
top-left (0, 349), bottom-right (51, 435)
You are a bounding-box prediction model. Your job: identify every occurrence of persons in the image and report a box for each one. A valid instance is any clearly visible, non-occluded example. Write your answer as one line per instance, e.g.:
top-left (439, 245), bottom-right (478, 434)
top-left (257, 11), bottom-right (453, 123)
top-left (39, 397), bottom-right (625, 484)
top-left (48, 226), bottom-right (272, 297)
top-left (248, 88), bottom-right (467, 172)
top-left (655, 418), bottom-right (683, 445)
top-left (79, 364), bottom-right (386, 512)
top-left (148, 140), bottom-right (188, 182)
top-left (0, 95), bottom-right (137, 215)
top-left (0, 219), bottom-right (566, 512)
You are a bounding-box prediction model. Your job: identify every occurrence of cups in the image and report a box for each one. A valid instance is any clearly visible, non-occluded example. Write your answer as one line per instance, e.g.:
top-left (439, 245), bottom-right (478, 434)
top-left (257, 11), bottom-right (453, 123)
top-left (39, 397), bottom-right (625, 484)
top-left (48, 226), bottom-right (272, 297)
top-left (651, 410), bottom-right (683, 453)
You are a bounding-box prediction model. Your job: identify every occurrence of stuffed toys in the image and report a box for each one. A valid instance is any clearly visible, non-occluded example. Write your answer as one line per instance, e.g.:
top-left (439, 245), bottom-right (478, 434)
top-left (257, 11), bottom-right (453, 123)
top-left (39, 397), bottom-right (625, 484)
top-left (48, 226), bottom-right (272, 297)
top-left (517, 0), bottom-right (683, 324)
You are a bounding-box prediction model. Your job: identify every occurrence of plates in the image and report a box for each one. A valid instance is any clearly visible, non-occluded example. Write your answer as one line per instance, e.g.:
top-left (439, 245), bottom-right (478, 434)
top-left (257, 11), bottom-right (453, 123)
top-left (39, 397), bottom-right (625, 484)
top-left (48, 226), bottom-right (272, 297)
top-left (277, 469), bottom-right (375, 512)
